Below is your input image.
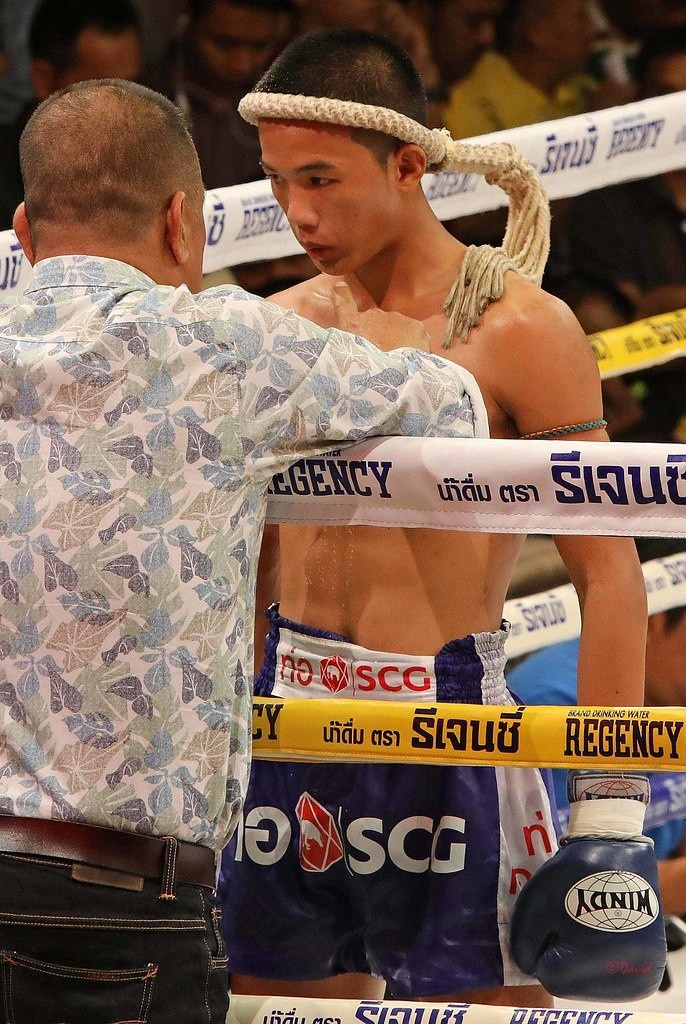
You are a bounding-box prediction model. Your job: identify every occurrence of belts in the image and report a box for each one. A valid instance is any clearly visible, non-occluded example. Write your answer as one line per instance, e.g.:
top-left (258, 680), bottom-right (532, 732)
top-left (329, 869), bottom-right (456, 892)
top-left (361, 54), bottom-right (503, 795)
top-left (0, 812), bottom-right (214, 891)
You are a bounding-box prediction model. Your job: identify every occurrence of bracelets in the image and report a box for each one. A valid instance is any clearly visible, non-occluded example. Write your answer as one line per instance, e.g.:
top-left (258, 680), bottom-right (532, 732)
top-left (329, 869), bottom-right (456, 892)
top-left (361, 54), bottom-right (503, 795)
top-left (559, 830), bottom-right (654, 848)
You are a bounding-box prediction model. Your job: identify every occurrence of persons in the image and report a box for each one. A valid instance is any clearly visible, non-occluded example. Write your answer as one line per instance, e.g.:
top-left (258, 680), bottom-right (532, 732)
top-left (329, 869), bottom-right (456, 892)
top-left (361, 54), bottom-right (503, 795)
top-left (0, 80), bottom-right (491, 1024)
top-left (215, 25), bottom-right (665, 1010)
top-left (0, 0), bottom-right (686, 996)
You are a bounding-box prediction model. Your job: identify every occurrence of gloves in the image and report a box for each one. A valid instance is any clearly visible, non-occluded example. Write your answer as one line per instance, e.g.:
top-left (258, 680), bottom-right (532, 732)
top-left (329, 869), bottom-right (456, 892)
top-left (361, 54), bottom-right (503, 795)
top-left (511, 770), bottom-right (669, 1002)
